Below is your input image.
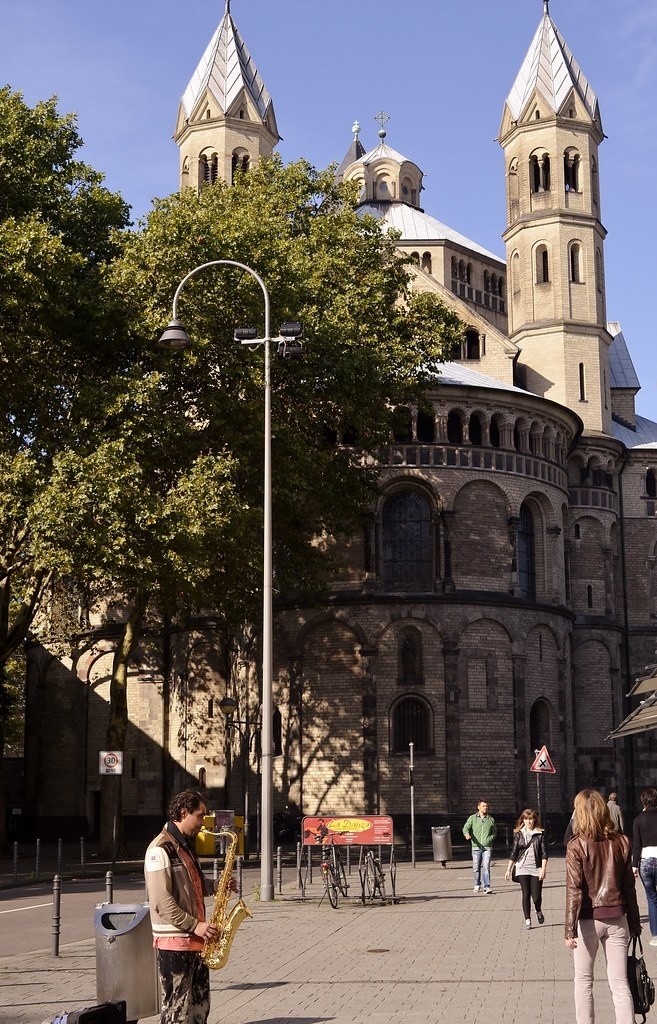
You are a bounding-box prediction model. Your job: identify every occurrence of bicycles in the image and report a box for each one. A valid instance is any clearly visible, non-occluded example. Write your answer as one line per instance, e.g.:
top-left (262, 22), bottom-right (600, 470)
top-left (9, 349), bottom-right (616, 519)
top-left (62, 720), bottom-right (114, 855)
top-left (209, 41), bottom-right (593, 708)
top-left (314, 829), bottom-right (351, 909)
top-left (362, 847), bottom-right (387, 900)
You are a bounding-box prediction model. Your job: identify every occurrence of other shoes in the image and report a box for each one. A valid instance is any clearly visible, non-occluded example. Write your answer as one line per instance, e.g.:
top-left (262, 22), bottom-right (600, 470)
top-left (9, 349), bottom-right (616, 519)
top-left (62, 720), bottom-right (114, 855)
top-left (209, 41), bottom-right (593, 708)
top-left (535, 910), bottom-right (544, 924)
top-left (525, 919), bottom-right (531, 930)
top-left (483, 887), bottom-right (493, 894)
top-left (473, 885), bottom-right (481, 892)
top-left (649, 937), bottom-right (657, 947)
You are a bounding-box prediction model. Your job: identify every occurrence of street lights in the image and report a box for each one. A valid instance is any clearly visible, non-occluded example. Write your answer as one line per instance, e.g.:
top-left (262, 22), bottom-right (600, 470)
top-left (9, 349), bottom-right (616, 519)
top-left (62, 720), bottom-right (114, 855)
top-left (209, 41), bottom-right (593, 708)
top-left (219, 695), bottom-right (277, 861)
top-left (154, 259), bottom-right (277, 905)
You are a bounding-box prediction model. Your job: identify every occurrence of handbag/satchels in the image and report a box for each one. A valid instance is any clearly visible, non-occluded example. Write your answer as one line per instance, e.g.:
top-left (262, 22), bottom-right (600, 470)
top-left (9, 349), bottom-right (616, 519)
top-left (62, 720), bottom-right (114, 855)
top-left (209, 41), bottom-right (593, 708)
top-left (511, 865), bottom-right (521, 883)
top-left (624, 936), bottom-right (656, 1024)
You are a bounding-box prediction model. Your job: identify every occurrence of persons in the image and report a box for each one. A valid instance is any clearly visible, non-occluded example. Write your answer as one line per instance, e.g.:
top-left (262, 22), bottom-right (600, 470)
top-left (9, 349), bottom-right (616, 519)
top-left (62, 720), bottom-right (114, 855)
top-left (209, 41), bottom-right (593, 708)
top-left (564, 789), bottom-right (644, 1024)
top-left (462, 800), bottom-right (497, 894)
top-left (632, 788), bottom-right (657, 946)
top-left (144, 790), bottom-right (240, 1024)
top-left (564, 792), bottom-right (625, 844)
top-left (504, 809), bottom-right (548, 930)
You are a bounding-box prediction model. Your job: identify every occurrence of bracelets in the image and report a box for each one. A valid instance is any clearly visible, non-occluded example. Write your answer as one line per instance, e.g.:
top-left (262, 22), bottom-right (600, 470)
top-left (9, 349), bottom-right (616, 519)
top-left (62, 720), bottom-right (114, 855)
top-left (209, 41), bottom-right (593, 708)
top-left (507, 869), bottom-right (511, 871)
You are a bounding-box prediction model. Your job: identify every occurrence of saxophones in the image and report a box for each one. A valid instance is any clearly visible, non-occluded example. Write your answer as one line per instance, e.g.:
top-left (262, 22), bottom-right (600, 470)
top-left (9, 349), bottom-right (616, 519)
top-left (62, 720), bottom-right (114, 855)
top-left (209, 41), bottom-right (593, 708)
top-left (198, 826), bottom-right (255, 970)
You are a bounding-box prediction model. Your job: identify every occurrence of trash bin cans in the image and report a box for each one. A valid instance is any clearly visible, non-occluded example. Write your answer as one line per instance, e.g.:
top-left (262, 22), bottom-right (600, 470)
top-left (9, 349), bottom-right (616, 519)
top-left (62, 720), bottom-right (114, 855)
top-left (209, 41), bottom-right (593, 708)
top-left (94, 902), bottom-right (160, 1021)
top-left (431, 826), bottom-right (452, 862)
top-left (220, 825), bottom-right (239, 854)
